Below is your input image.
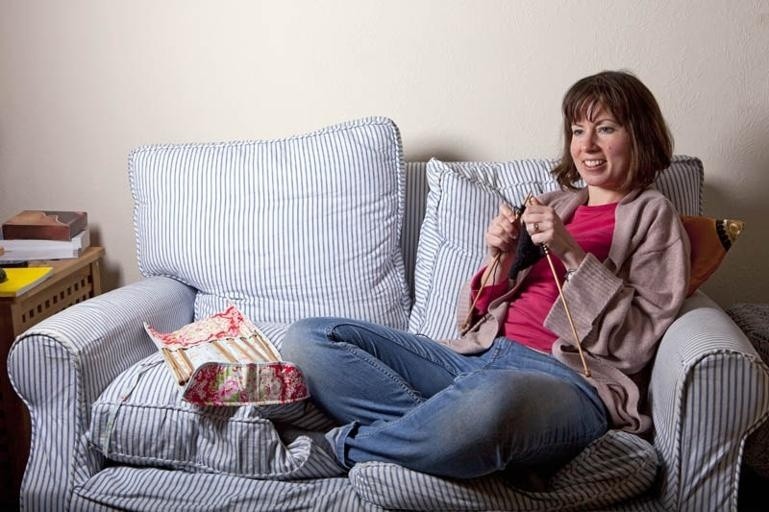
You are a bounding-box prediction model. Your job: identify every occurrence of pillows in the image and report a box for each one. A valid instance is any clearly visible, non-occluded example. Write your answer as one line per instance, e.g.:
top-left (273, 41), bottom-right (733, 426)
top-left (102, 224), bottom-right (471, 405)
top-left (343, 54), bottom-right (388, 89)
top-left (680, 218), bottom-right (742, 299)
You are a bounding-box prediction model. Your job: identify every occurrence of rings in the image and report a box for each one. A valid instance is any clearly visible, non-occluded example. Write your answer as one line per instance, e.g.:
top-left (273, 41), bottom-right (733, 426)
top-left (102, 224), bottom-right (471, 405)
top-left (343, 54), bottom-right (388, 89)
top-left (534, 223), bottom-right (539, 232)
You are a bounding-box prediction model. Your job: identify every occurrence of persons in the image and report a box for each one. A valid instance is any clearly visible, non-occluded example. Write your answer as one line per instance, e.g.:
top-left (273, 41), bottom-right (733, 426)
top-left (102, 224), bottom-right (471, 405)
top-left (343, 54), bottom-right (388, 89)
top-left (281, 71), bottom-right (692, 491)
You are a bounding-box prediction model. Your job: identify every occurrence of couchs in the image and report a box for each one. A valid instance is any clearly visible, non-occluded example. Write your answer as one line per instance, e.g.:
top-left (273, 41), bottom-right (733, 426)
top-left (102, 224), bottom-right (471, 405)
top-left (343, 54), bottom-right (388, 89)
top-left (1, 153), bottom-right (769, 512)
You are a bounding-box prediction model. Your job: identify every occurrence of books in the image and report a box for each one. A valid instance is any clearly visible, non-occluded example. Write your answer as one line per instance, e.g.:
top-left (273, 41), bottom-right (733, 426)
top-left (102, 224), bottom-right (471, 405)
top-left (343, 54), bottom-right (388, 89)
top-left (0, 210), bottom-right (90, 297)
